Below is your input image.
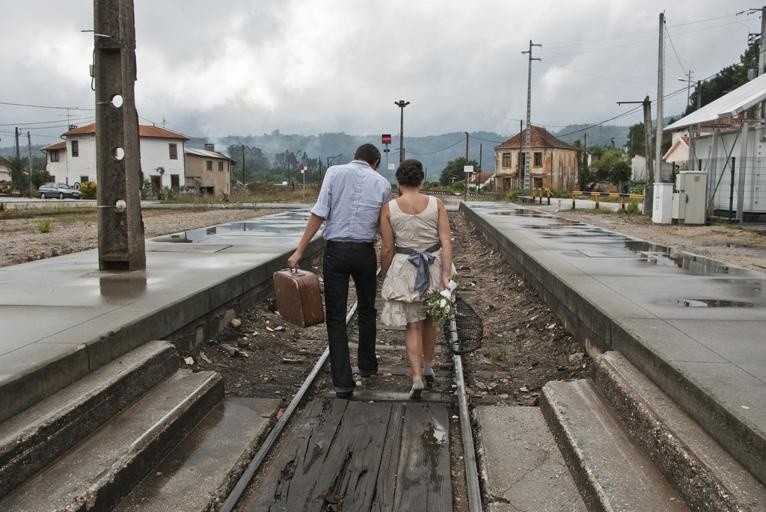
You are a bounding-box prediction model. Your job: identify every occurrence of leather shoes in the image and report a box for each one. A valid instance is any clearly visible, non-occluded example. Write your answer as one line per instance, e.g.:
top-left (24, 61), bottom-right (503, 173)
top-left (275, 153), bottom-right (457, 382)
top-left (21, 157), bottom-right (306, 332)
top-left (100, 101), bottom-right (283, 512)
top-left (335, 381), bottom-right (356, 399)
top-left (358, 365), bottom-right (378, 379)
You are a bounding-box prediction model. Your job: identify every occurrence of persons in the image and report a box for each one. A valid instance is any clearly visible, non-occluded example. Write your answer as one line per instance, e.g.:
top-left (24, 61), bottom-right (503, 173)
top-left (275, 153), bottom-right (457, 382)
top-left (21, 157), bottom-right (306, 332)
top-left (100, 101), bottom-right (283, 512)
top-left (378, 161), bottom-right (460, 400)
top-left (284, 142), bottom-right (391, 399)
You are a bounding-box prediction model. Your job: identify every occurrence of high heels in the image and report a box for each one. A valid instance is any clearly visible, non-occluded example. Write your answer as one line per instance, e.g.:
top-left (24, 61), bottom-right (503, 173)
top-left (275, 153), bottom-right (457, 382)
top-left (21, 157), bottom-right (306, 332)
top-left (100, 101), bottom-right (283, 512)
top-left (423, 367), bottom-right (437, 391)
top-left (408, 379), bottom-right (424, 402)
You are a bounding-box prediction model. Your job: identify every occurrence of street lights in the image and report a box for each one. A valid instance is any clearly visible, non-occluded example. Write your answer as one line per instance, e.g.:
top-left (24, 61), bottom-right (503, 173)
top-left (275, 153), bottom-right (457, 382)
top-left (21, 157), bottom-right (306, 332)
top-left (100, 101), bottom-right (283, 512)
top-left (395, 99), bottom-right (410, 166)
top-left (326, 153), bottom-right (343, 167)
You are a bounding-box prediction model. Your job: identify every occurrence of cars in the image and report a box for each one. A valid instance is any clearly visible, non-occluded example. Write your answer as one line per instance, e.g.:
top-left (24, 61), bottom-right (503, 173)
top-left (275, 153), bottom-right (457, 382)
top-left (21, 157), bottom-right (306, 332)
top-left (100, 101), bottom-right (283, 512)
top-left (38, 183), bottom-right (82, 199)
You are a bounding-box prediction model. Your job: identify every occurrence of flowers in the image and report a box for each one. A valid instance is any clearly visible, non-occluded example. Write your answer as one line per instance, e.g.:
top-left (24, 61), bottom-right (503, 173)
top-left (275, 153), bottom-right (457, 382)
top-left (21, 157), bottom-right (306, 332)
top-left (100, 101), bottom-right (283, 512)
top-left (415, 274), bottom-right (463, 322)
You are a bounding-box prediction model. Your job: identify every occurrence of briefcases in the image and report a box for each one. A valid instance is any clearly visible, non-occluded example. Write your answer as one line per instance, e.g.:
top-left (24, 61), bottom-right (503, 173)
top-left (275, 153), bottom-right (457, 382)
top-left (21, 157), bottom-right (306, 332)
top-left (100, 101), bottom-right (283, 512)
top-left (272, 265), bottom-right (325, 329)
